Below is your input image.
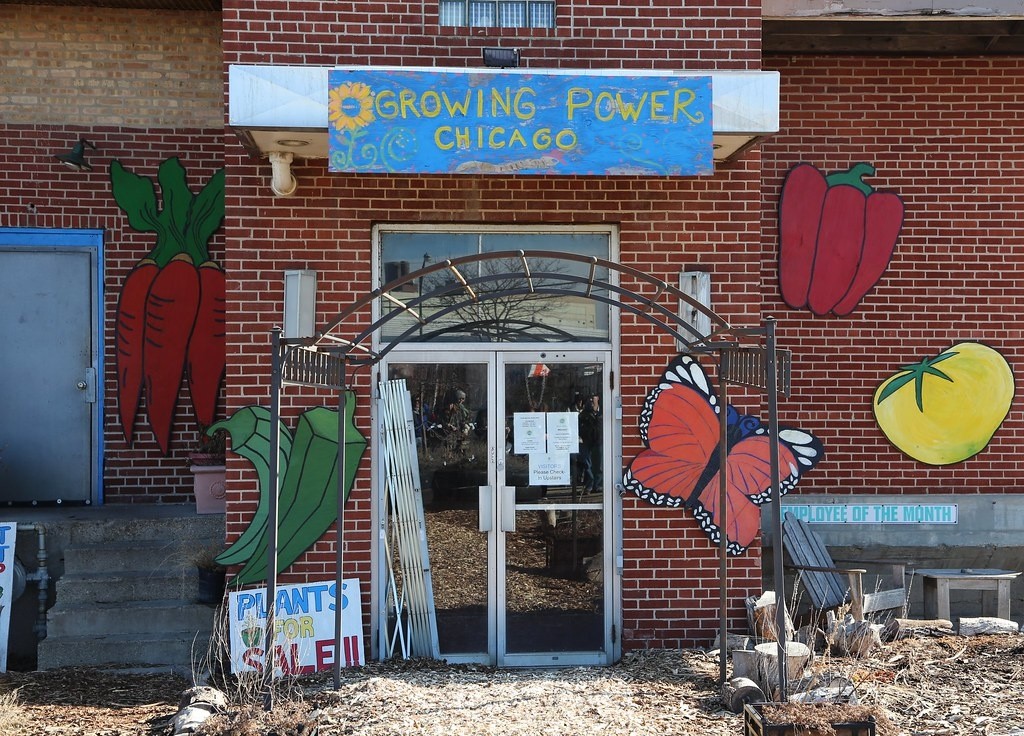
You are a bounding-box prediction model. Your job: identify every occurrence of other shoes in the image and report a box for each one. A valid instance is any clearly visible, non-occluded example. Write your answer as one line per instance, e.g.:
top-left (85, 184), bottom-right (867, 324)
top-left (586, 486), bottom-right (592, 491)
top-left (595, 487), bottom-right (603, 493)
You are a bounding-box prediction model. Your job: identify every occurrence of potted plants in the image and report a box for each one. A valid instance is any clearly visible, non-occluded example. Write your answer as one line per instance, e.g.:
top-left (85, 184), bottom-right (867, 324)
top-left (188, 424), bottom-right (225, 466)
top-left (167, 530), bottom-right (226, 604)
top-left (184, 422), bottom-right (225, 515)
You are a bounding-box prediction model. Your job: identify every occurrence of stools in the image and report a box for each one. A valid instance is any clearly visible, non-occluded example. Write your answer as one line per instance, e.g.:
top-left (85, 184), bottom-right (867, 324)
top-left (905, 568), bottom-right (1024, 621)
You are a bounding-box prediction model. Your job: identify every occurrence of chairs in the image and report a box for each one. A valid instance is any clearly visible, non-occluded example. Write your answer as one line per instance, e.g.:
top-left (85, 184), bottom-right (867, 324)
top-left (781, 512), bottom-right (906, 624)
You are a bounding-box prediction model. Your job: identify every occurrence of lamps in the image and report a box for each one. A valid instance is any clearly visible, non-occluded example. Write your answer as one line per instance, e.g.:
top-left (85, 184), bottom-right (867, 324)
top-left (53, 138), bottom-right (97, 173)
top-left (483, 46), bottom-right (521, 69)
top-left (284, 269), bottom-right (318, 363)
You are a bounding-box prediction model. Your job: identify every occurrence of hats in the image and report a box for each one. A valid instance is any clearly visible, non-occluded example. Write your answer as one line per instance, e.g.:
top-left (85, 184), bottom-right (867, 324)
top-left (574, 393), bottom-right (584, 400)
top-left (586, 390), bottom-right (598, 396)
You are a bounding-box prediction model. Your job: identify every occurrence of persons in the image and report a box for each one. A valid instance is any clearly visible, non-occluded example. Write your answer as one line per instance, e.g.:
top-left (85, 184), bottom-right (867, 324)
top-left (549, 387), bottom-right (606, 495)
top-left (413, 386), bottom-right (472, 463)
